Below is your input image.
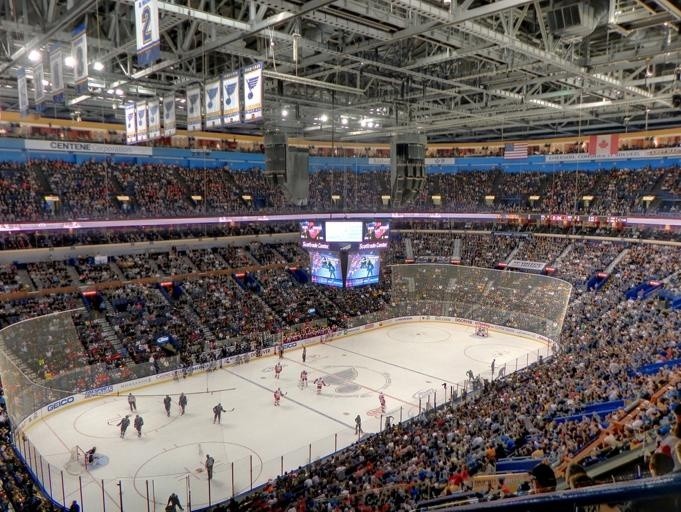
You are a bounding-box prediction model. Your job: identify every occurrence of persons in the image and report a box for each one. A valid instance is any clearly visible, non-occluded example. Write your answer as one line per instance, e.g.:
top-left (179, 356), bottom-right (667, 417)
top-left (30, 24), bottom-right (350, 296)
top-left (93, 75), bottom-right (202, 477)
top-left (0, 119), bottom-right (681, 512)
